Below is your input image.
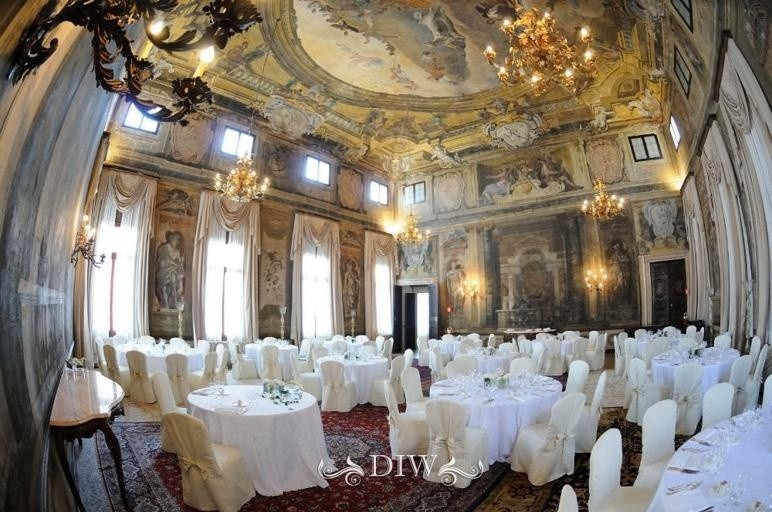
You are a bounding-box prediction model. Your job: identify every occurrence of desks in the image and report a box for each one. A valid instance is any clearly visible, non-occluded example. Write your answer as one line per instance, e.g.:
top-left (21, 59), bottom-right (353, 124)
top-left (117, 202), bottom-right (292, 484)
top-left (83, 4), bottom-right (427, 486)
top-left (48, 357), bottom-right (132, 511)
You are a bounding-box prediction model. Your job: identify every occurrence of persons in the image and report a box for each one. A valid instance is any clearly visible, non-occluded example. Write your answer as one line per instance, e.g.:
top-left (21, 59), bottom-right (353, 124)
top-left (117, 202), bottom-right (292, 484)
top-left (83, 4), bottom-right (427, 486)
top-left (481, 158), bottom-right (582, 203)
top-left (446, 259), bottom-right (466, 315)
top-left (344, 259), bottom-right (361, 314)
top-left (608, 242), bottom-right (631, 306)
top-left (226, 42), bottom-right (248, 61)
top-left (156, 231), bottom-right (185, 310)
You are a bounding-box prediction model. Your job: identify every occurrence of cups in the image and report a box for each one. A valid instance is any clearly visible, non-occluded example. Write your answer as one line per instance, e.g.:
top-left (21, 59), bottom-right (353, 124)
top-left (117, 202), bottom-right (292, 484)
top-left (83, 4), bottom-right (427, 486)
top-left (702, 400), bottom-right (772, 512)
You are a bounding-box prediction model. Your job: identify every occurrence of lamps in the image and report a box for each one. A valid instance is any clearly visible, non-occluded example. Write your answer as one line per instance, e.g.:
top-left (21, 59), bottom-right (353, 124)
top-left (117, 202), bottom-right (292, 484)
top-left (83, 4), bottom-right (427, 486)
top-left (577, 175), bottom-right (625, 222)
top-left (6, 0), bottom-right (263, 127)
top-left (68, 214), bottom-right (107, 269)
top-left (386, 206), bottom-right (431, 246)
top-left (473, 1), bottom-right (599, 100)
top-left (212, 16), bottom-right (284, 206)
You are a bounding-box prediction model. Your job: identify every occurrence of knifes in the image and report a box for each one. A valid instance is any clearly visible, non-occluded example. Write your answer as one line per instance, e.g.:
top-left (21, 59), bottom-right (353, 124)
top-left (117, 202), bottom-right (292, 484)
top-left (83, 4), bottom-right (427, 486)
top-left (668, 466), bottom-right (699, 476)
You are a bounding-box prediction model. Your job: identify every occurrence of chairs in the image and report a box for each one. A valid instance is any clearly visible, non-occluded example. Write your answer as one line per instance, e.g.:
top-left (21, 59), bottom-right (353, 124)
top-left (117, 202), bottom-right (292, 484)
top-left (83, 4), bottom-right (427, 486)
top-left (88, 328), bottom-right (772, 512)
